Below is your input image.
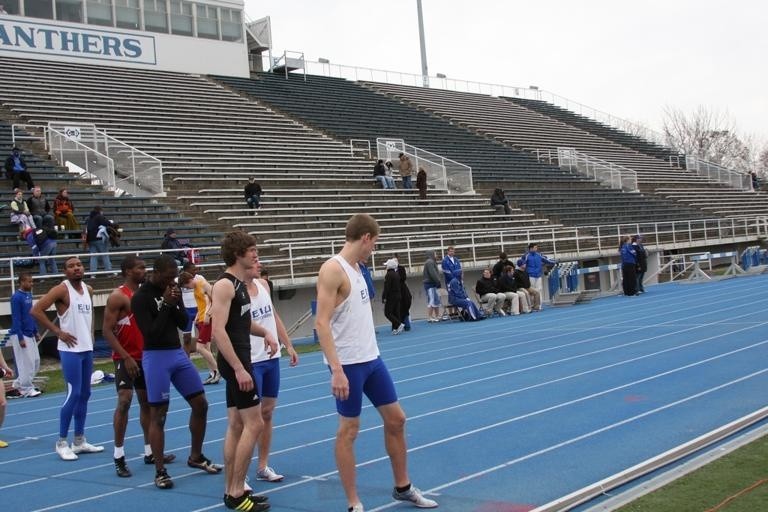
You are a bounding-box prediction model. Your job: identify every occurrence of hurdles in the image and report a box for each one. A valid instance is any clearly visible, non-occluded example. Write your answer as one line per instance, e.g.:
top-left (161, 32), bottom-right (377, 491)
top-left (0, 328), bottom-right (49, 392)
top-left (688, 252), bottom-right (746, 281)
top-left (740, 246), bottom-right (768, 275)
top-left (546, 261), bottom-right (623, 305)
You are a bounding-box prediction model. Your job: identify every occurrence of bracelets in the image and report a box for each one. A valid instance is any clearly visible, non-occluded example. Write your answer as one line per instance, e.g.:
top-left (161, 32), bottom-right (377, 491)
top-left (161, 301), bottom-right (172, 309)
top-left (56, 327), bottom-right (60, 335)
top-left (3, 364), bottom-right (7, 369)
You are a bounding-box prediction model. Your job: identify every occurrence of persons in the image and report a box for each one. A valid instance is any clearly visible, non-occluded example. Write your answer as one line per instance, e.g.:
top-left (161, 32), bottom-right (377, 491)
top-left (749, 171), bottom-right (758, 188)
top-left (161, 228), bottom-right (190, 265)
top-left (384, 158), bottom-right (398, 190)
top-left (5, 147), bottom-right (36, 191)
top-left (490, 187), bottom-right (513, 220)
top-left (29, 256), bottom-right (106, 460)
top-left (11, 186), bottom-right (123, 280)
top-left (0, 350), bottom-right (14, 447)
top-left (130, 255), bottom-right (222, 488)
top-left (212, 230), bottom-right (279, 511)
top-left (176, 262), bottom-right (207, 359)
top-left (178, 271), bottom-right (221, 385)
top-left (416, 167), bottom-right (427, 203)
top-left (618, 235), bottom-right (638, 296)
top-left (102, 255), bottom-right (176, 477)
top-left (380, 234), bottom-right (557, 334)
top-left (317, 213), bottom-right (437, 512)
top-left (243, 255), bottom-right (300, 491)
top-left (398, 152), bottom-right (413, 188)
top-left (261, 269), bottom-right (274, 304)
top-left (633, 235), bottom-right (649, 294)
top-left (10, 274), bottom-right (44, 396)
top-left (374, 160), bottom-right (394, 189)
top-left (244, 176), bottom-right (262, 216)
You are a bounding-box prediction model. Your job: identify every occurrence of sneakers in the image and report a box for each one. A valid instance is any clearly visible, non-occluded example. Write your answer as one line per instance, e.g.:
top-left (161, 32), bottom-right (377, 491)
top-left (144, 454), bottom-right (176, 464)
top-left (155, 469), bottom-right (173, 490)
top-left (187, 455), bottom-right (222, 474)
top-left (256, 466), bottom-right (284, 482)
top-left (56, 441), bottom-right (78, 460)
top-left (113, 456), bottom-right (131, 477)
top-left (224, 481), bottom-right (270, 511)
top-left (0, 441), bottom-right (8, 448)
top-left (72, 438), bottom-right (104, 453)
top-left (390, 304), bottom-right (542, 335)
top-left (202, 369), bottom-right (221, 385)
top-left (29, 390), bottom-right (40, 398)
top-left (392, 485), bottom-right (439, 509)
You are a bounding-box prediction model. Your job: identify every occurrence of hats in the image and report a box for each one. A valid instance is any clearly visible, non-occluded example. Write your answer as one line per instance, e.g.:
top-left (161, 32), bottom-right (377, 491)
top-left (383, 259), bottom-right (398, 268)
